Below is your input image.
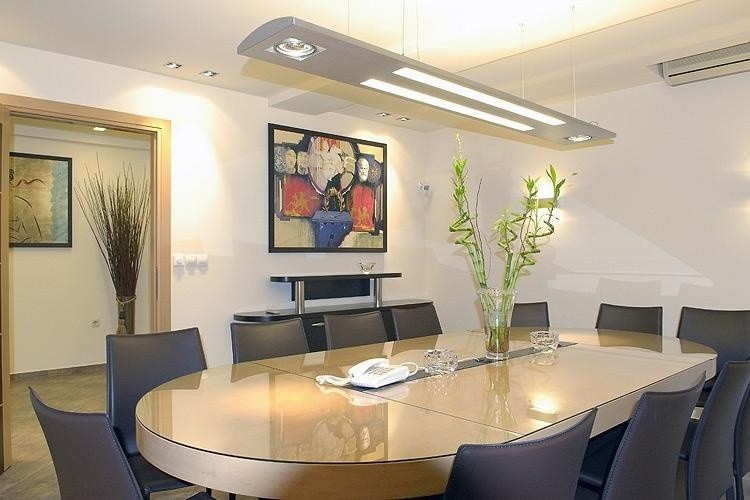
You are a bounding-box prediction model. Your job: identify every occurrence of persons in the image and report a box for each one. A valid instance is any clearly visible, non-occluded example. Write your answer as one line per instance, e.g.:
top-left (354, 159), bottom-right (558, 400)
top-left (350, 157), bottom-right (374, 196)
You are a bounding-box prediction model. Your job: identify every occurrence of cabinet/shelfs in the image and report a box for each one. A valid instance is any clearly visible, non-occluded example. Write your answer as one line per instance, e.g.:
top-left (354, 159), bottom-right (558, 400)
top-left (234, 273), bottom-right (435, 353)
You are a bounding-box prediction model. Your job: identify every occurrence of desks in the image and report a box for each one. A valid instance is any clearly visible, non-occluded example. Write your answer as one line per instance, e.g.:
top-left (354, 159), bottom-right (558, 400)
top-left (133, 326), bottom-right (716, 499)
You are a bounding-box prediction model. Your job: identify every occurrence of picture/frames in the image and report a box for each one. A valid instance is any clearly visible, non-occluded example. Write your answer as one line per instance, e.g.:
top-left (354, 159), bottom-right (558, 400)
top-left (266, 121), bottom-right (389, 252)
top-left (8, 150), bottom-right (75, 247)
top-left (267, 368), bottom-right (390, 471)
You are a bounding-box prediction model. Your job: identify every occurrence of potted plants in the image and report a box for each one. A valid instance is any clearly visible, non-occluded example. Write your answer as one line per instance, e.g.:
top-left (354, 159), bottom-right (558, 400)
top-left (444, 131), bottom-right (571, 360)
top-left (470, 360), bottom-right (524, 445)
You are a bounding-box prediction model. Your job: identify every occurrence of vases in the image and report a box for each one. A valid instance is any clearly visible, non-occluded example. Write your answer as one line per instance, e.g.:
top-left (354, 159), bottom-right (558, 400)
top-left (116, 296), bottom-right (139, 336)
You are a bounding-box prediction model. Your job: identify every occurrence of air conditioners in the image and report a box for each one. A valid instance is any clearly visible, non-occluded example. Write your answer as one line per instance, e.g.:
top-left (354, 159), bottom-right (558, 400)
top-left (659, 41), bottom-right (750, 88)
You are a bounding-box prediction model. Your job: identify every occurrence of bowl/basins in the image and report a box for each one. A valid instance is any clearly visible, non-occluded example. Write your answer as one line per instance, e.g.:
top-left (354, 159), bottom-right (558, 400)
top-left (529, 331), bottom-right (560, 353)
top-left (424, 377), bottom-right (459, 399)
top-left (422, 349), bottom-right (459, 376)
top-left (529, 354), bottom-right (558, 366)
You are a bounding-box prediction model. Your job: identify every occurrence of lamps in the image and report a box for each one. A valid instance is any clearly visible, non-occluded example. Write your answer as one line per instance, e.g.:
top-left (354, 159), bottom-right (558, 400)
top-left (237, 0), bottom-right (615, 148)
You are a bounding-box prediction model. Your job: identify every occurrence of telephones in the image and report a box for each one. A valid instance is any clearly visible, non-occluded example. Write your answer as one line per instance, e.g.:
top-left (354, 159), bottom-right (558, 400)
top-left (348, 358), bottom-right (410, 389)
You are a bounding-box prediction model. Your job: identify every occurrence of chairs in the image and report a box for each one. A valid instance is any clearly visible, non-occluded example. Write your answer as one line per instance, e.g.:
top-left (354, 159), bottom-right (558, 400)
top-left (23, 386), bottom-right (220, 500)
top-left (390, 305), bottom-right (445, 340)
top-left (574, 368), bottom-right (710, 499)
top-left (675, 305), bottom-right (750, 407)
top-left (442, 407), bottom-right (600, 498)
top-left (675, 359), bottom-right (749, 499)
top-left (509, 299), bottom-right (550, 327)
top-left (322, 310), bottom-right (389, 351)
top-left (227, 316), bottom-right (310, 365)
top-left (101, 324), bottom-right (208, 493)
top-left (594, 303), bottom-right (664, 335)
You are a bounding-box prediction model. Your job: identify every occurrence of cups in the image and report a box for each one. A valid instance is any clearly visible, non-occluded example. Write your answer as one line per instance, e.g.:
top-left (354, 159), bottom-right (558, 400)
top-left (359, 261), bottom-right (376, 274)
